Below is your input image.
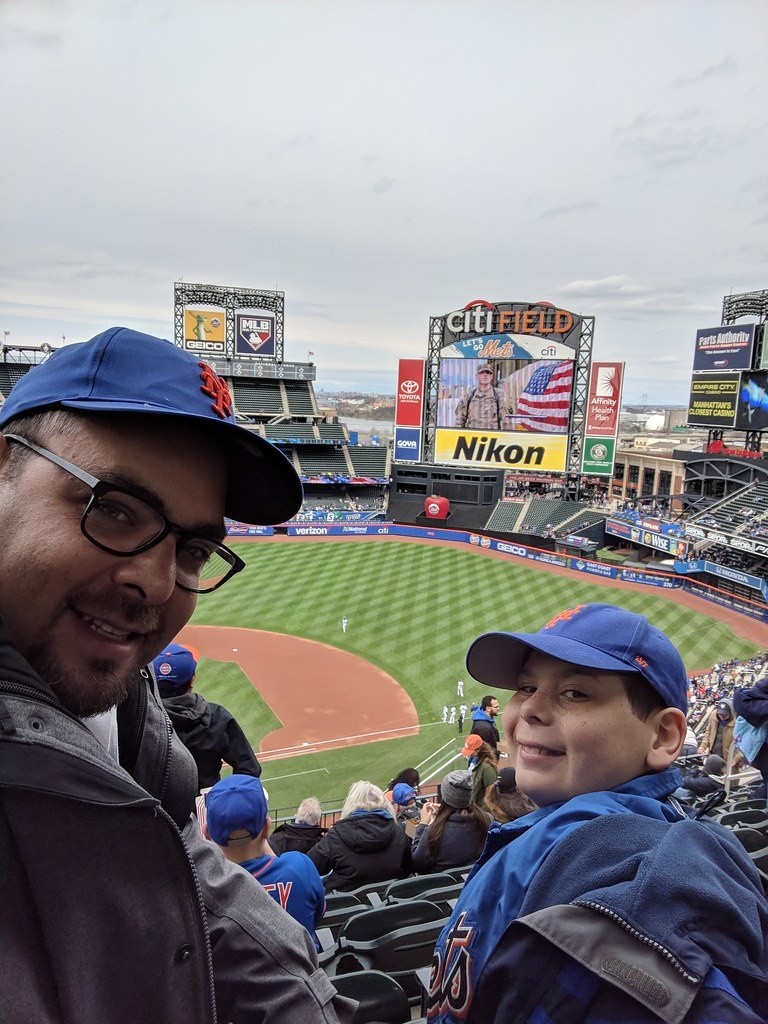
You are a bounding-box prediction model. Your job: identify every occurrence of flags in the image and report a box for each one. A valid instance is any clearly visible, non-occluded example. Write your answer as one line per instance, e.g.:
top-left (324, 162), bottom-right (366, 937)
top-left (515, 362), bottom-right (571, 432)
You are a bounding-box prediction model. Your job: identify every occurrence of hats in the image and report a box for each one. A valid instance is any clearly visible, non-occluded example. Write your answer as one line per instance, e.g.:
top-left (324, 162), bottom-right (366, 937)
top-left (461, 733), bottom-right (482, 756)
top-left (497, 767), bottom-right (516, 789)
top-left (477, 364), bottom-right (494, 374)
top-left (0, 326), bottom-right (304, 529)
top-left (465, 603), bottom-right (687, 718)
top-left (205, 774), bottom-right (267, 846)
top-left (153, 643), bottom-right (201, 690)
top-left (441, 770), bottom-right (474, 808)
top-left (702, 754), bottom-right (727, 776)
top-left (716, 702), bottom-right (729, 715)
top-left (393, 784), bottom-right (417, 807)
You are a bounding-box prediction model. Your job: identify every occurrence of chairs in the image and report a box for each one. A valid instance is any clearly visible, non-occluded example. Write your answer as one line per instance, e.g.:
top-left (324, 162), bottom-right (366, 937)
top-left (0, 362), bottom-right (40, 399)
top-left (694, 777), bottom-right (768, 890)
top-left (312, 865), bottom-right (477, 1024)
top-left (231, 380), bottom-right (768, 605)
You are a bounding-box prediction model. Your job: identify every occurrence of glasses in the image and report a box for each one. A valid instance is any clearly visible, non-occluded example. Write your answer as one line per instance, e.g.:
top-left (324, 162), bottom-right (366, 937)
top-left (4, 432), bottom-right (246, 594)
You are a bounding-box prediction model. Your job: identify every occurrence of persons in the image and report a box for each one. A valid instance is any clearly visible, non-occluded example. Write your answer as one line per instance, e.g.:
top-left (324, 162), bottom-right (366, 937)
top-left (0, 646), bottom-right (768, 1024)
top-left (410, 600), bottom-right (767, 1024)
top-left (0, 327), bottom-right (409, 1024)
top-left (454, 362), bottom-right (514, 431)
